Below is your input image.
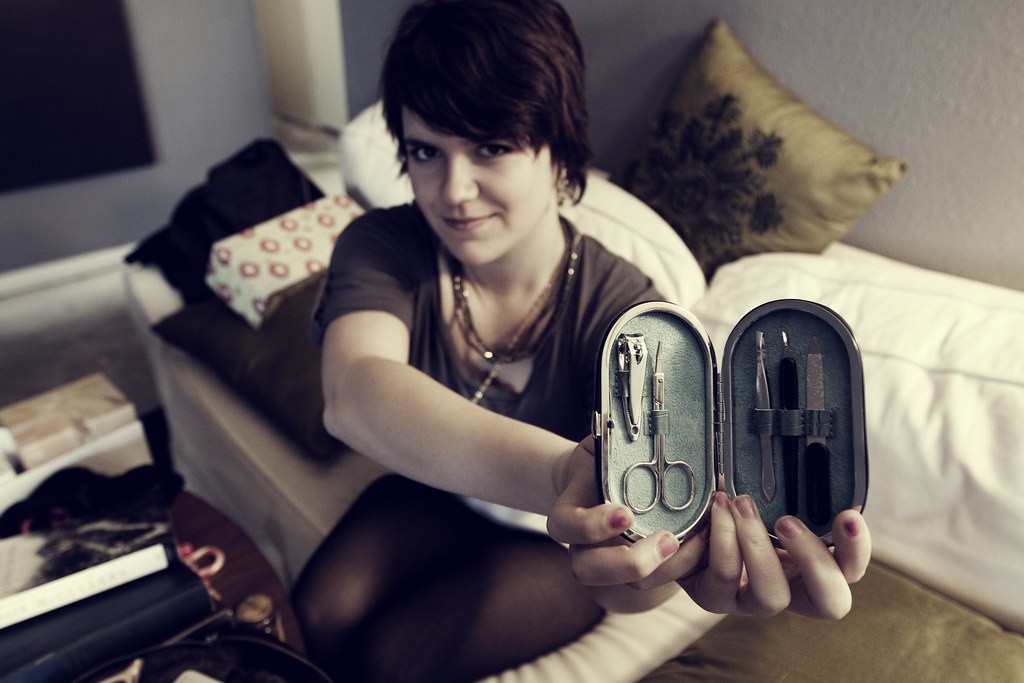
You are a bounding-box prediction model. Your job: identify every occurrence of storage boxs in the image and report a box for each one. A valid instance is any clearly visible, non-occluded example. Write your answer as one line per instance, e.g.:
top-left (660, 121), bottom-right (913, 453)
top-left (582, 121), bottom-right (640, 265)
top-left (204, 193), bottom-right (368, 326)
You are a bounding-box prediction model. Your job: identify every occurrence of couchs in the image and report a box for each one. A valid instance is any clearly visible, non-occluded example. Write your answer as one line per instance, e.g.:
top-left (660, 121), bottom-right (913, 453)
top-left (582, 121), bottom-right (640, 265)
top-left (121, 96), bottom-right (1024, 683)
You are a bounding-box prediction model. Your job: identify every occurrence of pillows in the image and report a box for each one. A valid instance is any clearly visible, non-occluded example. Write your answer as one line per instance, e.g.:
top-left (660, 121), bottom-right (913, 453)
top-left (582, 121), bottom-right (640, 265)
top-left (144, 275), bottom-right (351, 461)
top-left (634, 17), bottom-right (909, 289)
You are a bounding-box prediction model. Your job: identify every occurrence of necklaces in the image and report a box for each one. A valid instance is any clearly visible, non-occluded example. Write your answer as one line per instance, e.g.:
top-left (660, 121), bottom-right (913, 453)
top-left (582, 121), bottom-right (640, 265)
top-left (445, 229), bottom-right (583, 404)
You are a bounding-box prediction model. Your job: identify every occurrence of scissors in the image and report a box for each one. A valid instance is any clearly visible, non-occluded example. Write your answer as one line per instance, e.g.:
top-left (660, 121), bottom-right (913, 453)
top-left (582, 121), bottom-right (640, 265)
top-left (620, 340), bottom-right (697, 516)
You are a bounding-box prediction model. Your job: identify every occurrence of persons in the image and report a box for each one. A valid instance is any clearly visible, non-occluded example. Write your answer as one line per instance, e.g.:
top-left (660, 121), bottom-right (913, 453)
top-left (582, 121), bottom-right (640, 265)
top-left (288, 0), bottom-right (872, 683)
